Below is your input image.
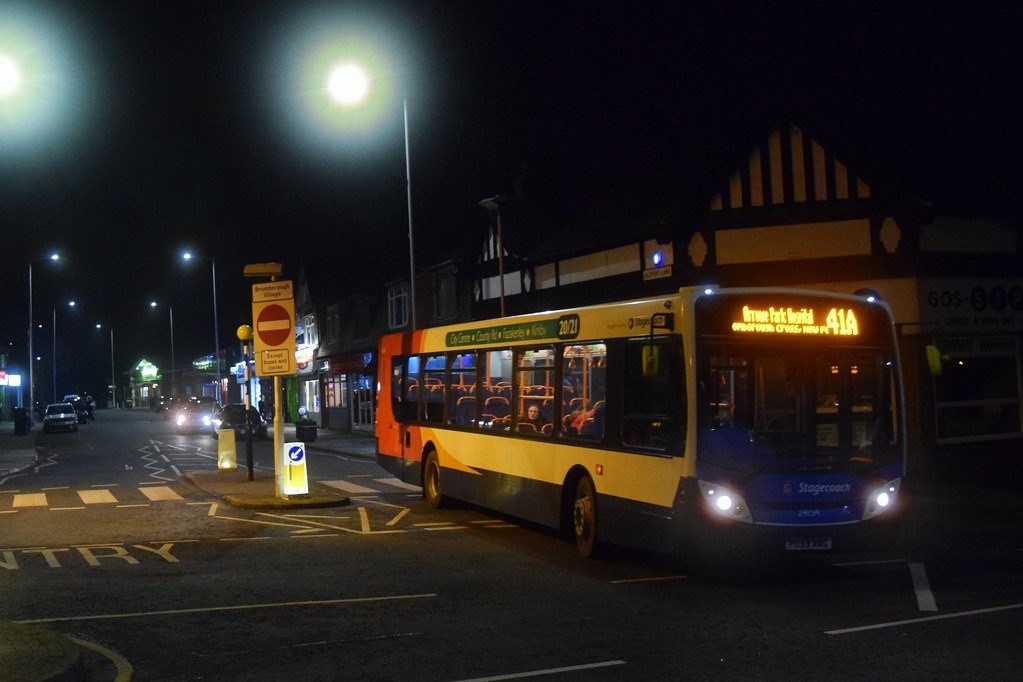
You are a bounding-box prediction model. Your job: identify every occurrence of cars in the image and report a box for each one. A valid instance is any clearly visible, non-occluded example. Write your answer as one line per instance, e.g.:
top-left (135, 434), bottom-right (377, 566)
top-left (155, 394), bottom-right (222, 433)
top-left (62, 392), bottom-right (96, 425)
top-left (42, 402), bottom-right (80, 433)
top-left (211, 402), bottom-right (266, 440)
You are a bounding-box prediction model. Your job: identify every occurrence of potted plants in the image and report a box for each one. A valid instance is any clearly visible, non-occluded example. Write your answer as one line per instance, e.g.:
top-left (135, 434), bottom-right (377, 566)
top-left (296, 420), bottom-right (319, 442)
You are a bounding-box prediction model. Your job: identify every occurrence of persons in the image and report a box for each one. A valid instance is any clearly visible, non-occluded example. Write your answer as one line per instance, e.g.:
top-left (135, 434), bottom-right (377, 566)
top-left (518, 402), bottom-right (580, 433)
top-left (86, 396), bottom-right (96, 421)
top-left (258, 394), bottom-right (267, 423)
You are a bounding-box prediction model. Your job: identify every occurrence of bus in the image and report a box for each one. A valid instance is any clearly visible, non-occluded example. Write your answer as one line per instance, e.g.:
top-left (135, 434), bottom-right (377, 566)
top-left (374, 283), bottom-right (953, 575)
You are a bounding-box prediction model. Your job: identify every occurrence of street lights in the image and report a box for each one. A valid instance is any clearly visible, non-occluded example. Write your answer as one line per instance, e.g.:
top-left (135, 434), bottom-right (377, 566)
top-left (183, 253), bottom-right (223, 407)
top-left (302, 38), bottom-right (418, 330)
top-left (477, 195), bottom-right (506, 317)
top-left (28, 253), bottom-right (62, 432)
top-left (96, 321), bottom-right (116, 409)
top-left (51, 301), bottom-right (78, 404)
top-left (150, 301), bottom-right (175, 396)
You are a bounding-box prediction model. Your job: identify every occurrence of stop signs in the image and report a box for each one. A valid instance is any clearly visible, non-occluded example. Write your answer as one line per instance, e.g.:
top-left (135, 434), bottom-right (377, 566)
top-left (250, 301), bottom-right (297, 353)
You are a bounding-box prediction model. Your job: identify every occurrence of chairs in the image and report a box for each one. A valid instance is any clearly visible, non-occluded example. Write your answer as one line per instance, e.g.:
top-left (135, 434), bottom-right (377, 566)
top-left (396, 377), bottom-right (605, 438)
